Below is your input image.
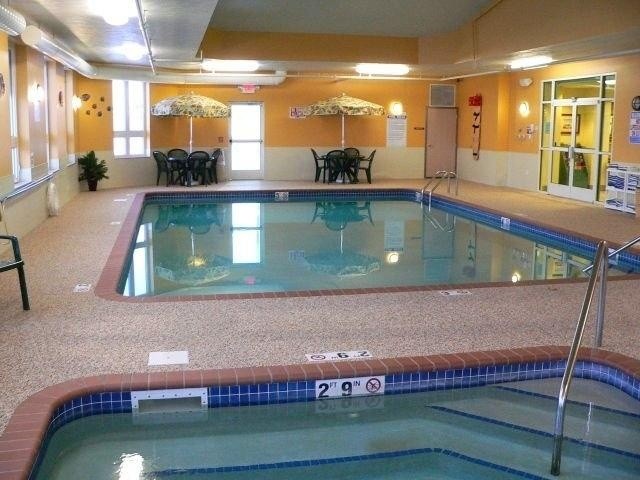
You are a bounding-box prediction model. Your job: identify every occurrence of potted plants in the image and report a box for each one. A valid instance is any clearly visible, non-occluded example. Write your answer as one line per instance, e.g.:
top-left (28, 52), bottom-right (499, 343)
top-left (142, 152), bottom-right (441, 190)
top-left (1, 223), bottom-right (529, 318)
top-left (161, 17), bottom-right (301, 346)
top-left (78, 148), bottom-right (111, 191)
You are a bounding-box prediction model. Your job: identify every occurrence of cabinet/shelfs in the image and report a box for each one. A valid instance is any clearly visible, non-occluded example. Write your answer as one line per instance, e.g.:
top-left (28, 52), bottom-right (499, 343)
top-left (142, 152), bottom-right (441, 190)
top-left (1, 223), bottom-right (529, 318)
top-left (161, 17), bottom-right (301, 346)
top-left (604, 161), bottom-right (640, 215)
top-left (560, 142), bottom-right (589, 189)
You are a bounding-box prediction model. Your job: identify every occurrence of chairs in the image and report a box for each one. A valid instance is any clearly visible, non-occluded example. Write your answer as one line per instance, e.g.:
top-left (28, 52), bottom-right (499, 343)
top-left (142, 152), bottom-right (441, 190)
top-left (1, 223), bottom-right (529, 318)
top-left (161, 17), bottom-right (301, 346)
top-left (1, 233), bottom-right (33, 313)
top-left (149, 201), bottom-right (223, 237)
top-left (308, 200), bottom-right (377, 233)
top-left (309, 145), bottom-right (378, 184)
top-left (150, 146), bottom-right (223, 189)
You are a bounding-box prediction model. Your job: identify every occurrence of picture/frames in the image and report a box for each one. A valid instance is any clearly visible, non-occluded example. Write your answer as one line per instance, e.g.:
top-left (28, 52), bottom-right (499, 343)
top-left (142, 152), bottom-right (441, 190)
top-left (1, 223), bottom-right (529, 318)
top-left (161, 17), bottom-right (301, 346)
top-left (552, 257), bottom-right (572, 277)
top-left (560, 114), bottom-right (581, 134)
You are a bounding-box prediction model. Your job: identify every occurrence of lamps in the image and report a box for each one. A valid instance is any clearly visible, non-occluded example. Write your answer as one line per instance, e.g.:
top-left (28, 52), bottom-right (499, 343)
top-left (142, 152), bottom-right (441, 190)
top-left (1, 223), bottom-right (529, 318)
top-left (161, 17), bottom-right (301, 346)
top-left (631, 95), bottom-right (640, 112)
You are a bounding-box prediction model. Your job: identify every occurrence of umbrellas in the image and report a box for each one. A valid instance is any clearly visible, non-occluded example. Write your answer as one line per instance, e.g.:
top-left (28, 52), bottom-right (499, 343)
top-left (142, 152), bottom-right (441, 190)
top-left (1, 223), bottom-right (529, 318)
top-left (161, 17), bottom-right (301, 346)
top-left (150, 94), bottom-right (230, 153)
top-left (303, 92), bottom-right (385, 151)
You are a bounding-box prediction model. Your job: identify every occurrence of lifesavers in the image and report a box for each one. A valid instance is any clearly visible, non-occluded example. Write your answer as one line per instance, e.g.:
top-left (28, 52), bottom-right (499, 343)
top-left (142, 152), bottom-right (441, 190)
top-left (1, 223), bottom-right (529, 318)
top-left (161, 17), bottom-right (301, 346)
top-left (47, 184), bottom-right (61, 217)
